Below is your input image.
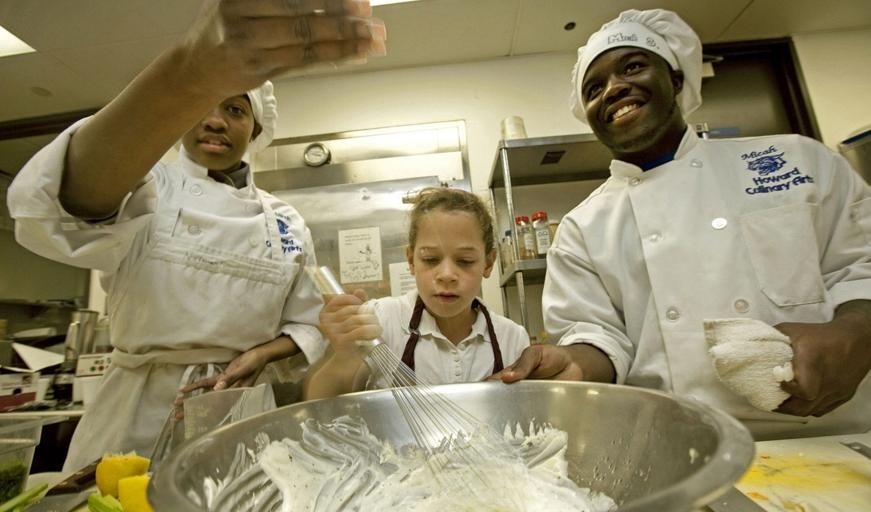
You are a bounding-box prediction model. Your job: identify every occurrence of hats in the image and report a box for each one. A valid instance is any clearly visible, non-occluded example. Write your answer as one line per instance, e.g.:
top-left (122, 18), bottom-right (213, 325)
top-left (248, 80), bottom-right (276, 157)
top-left (569, 9), bottom-right (702, 122)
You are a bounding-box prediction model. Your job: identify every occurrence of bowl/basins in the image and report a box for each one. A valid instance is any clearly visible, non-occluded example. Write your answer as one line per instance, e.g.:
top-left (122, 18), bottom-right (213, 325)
top-left (146, 380), bottom-right (755, 511)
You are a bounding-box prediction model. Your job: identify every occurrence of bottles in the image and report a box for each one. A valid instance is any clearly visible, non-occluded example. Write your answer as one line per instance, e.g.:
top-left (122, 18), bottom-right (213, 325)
top-left (501, 210), bottom-right (553, 260)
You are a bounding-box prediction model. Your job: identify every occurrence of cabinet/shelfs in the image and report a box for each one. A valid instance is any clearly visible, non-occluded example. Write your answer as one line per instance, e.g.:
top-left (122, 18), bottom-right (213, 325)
top-left (487, 121), bottom-right (710, 349)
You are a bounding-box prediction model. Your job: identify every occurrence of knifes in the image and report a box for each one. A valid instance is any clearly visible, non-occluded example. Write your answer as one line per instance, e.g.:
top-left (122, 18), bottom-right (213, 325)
top-left (18, 456), bottom-right (102, 512)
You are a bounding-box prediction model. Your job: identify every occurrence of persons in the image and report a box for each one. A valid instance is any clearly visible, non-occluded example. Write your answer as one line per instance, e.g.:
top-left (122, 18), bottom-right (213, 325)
top-left (487, 8), bottom-right (871, 441)
top-left (6, 3), bottom-right (387, 474)
top-left (306, 187), bottom-right (528, 408)
